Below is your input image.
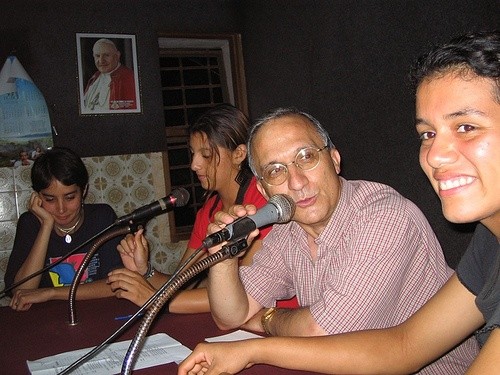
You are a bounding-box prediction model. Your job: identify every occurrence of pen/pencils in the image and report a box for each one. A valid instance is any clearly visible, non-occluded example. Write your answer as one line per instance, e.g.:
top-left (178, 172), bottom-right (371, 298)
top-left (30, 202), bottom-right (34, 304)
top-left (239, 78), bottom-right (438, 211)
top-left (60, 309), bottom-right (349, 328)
top-left (114, 312), bottom-right (144, 320)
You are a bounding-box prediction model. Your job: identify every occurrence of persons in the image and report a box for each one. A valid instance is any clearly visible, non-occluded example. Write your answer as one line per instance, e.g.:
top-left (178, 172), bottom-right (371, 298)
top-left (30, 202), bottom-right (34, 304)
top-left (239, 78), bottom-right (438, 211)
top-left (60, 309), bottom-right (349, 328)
top-left (206, 107), bottom-right (479, 375)
top-left (105, 104), bottom-right (302, 314)
top-left (175, 29), bottom-right (500, 375)
top-left (6, 147), bottom-right (127, 312)
top-left (84, 38), bottom-right (137, 109)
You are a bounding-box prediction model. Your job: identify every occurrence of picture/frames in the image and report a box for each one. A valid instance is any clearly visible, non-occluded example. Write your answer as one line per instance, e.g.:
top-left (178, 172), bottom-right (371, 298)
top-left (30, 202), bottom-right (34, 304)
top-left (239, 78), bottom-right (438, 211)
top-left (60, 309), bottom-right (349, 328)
top-left (76, 34), bottom-right (142, 116)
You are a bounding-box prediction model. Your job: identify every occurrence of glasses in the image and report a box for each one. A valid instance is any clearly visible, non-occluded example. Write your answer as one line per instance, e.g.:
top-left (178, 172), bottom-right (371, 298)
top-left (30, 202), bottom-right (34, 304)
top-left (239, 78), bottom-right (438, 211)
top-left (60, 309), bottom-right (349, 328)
top-left (258, 144), bottom-right (330, 186)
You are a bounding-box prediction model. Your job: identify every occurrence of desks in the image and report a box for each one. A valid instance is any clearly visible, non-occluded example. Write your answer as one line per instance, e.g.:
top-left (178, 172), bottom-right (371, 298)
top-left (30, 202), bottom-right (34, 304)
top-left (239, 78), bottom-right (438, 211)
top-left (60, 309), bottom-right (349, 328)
top-left (0, 290), bottom-right (329, 375)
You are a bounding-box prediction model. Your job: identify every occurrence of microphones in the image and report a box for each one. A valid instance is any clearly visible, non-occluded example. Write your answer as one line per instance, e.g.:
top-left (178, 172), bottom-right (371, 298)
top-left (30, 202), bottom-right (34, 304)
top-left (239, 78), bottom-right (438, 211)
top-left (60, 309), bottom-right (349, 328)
top-left (114, 188), bottom-right (189, 225)
top-left (203, 194), bottom-right (296, 249)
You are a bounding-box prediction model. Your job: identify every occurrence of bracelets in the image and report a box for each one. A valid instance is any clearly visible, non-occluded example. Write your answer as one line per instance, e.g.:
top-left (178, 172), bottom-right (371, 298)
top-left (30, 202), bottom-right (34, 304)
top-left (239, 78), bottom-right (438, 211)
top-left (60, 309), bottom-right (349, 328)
top-left (142, 262), bottom-right (155, 279)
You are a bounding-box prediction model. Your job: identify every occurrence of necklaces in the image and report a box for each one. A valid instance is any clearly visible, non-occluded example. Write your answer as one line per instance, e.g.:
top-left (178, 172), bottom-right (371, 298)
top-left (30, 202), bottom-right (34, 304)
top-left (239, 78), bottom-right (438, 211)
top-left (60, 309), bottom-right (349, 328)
top-left (54, 213), bottom-right (82, 244)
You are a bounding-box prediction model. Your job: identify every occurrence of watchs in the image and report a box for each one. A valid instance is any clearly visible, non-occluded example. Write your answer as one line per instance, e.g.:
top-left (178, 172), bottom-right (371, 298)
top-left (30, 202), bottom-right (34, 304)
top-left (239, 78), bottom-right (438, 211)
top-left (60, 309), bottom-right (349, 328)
top-left (260, 307), bottom-right (278, 336)
